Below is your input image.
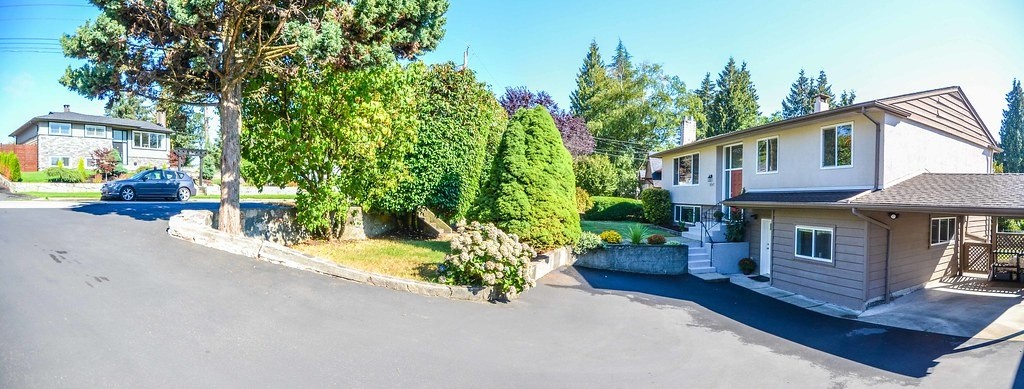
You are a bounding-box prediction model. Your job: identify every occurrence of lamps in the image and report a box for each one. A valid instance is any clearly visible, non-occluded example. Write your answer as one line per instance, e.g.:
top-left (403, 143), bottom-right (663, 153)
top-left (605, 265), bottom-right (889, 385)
top-left (889, 212), bottom-right (899, 219)
top-left (707, 174), bottom-right (713, 186)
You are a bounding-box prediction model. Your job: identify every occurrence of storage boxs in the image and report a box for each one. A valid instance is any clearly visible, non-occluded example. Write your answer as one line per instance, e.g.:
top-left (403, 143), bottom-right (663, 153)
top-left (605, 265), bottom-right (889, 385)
top-left (996, 272), bottom-right (1010, 280)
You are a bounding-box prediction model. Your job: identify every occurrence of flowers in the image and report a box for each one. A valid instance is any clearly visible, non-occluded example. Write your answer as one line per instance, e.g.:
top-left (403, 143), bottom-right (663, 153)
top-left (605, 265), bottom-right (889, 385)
top-left (738, 258), bottom-right (756, 271)
top-left (647, 234), bottom-right (665, 244)
top-left (599, 229), bottom-right (623, 244)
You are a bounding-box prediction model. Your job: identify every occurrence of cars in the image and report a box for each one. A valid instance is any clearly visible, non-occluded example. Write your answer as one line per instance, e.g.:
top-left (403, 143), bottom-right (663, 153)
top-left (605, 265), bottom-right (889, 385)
top-left (100, 168), bottom-right (197, 201)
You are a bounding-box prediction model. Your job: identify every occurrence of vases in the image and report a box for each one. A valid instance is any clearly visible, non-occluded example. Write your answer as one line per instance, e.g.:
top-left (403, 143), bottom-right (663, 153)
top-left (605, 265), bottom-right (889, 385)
top-left (743, 270), bottom-right (750, 275)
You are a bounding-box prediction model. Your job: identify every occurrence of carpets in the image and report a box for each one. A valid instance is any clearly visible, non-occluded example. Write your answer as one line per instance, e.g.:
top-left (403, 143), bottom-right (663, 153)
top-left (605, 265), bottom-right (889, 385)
top-left (749, 275), bottom-right (770, 282)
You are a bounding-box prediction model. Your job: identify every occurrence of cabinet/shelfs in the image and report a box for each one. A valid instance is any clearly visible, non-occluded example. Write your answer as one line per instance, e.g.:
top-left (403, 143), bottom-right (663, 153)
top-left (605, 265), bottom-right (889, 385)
top-left (992, 249), bottom-right (1020, 282)
